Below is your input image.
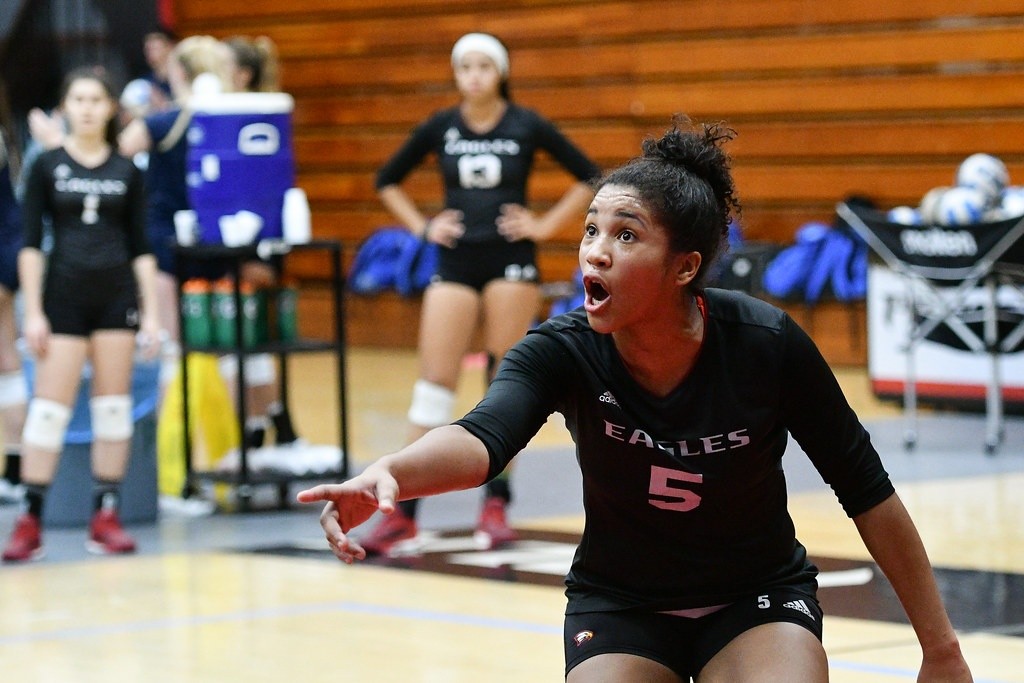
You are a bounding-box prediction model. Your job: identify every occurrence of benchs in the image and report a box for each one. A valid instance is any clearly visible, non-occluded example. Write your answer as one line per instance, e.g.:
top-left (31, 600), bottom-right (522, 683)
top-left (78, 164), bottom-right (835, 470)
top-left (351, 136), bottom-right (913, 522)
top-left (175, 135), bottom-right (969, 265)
top-left (160, 0), bottom-right (1024, 282)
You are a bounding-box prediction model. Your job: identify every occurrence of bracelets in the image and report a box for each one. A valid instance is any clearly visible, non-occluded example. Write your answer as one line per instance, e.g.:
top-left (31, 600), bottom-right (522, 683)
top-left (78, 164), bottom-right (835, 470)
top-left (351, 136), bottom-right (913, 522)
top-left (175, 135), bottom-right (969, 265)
top-left (422, 217), bottom-right (434, 242)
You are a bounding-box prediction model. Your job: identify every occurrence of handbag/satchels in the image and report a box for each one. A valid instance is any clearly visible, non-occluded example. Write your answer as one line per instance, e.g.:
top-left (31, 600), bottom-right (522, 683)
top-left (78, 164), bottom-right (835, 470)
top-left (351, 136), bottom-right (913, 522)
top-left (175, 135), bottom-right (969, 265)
top-left (348, 227), bottom-right (441, 296)
top-left (762, 197), bottom-right (875, 305)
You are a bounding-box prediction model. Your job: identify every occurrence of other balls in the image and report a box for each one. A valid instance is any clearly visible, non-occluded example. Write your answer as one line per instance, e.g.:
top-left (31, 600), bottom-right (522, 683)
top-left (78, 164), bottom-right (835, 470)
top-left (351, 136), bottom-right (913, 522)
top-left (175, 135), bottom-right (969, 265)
top-left (888, 153), bottom-right (1023, 226)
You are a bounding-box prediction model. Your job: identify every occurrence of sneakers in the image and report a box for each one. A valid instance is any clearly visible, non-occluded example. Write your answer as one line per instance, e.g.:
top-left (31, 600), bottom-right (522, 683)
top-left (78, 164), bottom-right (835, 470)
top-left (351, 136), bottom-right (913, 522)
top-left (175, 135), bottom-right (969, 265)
top-left (357, 505), bottom-right (418, 554)
top-left (87, 512), bottom-right (135, 552)
top-left (2, 513), bottom-right (45, 564)
top-left (477, 497), bottom-right (520, 548)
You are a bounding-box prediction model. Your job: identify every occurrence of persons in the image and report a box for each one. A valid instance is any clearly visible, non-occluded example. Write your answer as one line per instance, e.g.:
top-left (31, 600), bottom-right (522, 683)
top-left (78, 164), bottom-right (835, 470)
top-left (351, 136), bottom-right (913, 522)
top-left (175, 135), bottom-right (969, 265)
top-left (0, 26), bottom-right (604, 562)
top-left (296, 112), bottom-right (975, 683)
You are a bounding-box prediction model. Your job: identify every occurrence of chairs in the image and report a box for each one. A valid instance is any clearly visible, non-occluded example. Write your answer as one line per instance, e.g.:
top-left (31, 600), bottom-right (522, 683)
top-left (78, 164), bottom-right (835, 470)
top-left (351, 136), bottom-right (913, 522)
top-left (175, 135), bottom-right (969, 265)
top-left (770, 195), bottom-right (1024, 456)
